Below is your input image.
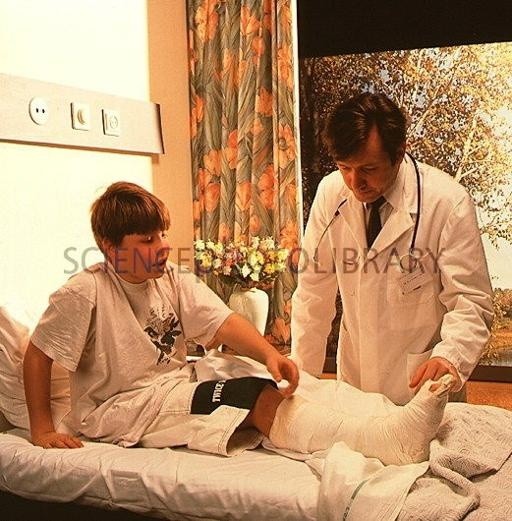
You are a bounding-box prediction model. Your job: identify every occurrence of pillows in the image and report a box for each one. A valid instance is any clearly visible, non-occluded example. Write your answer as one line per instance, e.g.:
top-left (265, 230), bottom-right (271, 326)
top-left (0, 303), bottom-right (74, 438)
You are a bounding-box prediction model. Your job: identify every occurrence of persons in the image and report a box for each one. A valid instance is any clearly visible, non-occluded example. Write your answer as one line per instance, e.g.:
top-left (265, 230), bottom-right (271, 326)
top-left (290, 91), bottom-right (495, 406)
top-left (22, 182), bottom-right (456, 467)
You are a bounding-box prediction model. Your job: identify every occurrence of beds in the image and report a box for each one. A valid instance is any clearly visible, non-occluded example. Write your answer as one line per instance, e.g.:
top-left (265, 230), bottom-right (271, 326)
top-left (5, 368), bottom-right (511, 521)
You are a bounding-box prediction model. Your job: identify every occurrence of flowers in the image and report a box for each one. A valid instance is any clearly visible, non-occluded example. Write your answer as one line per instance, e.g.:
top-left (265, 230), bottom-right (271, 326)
top-left (192, 235), bottom-right (289, 289)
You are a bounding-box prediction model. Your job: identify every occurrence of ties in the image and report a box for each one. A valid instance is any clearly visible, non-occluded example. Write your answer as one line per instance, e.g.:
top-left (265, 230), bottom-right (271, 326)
top-left (365, 196), bottom-right (387, 249)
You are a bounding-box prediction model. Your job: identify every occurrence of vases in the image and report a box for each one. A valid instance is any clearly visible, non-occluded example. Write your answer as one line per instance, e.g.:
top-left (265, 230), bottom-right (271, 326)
top-left (229, 287), bottom-right (270, 343)
